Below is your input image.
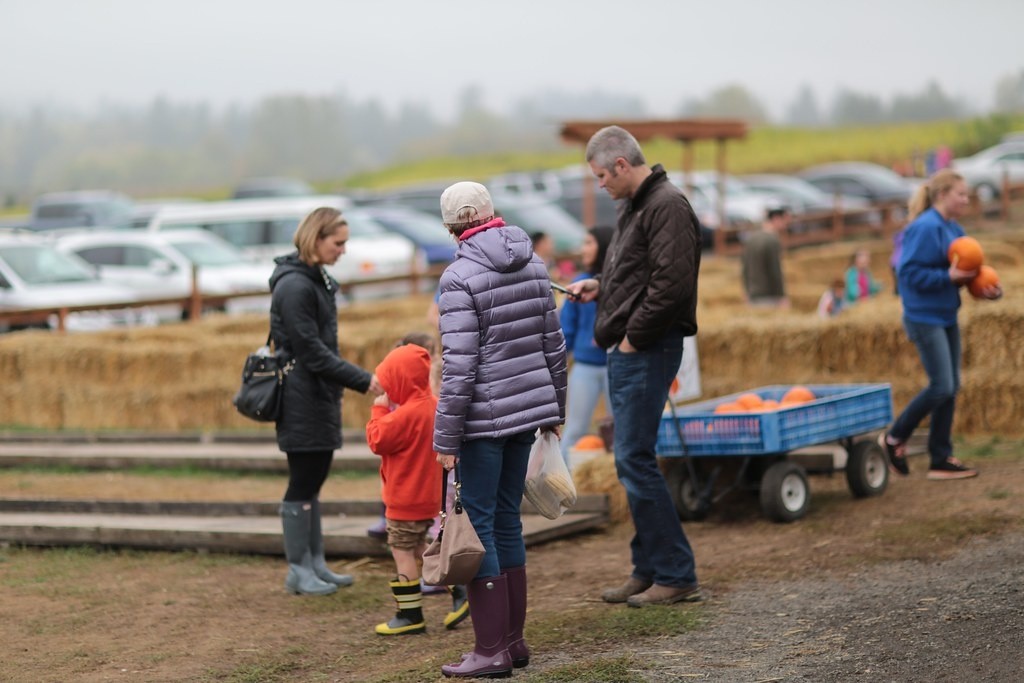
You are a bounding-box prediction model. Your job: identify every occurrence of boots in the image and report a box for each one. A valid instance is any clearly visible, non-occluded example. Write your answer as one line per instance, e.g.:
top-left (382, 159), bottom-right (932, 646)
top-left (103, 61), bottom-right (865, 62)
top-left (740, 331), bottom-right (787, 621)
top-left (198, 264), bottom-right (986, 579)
top-left (279, 501), bottom-right (339, 596)
top-left (310, 501), bottom-right (353, 586)
top-left (373, 574), bottom-right (426, 634)
top-left (442, 575), bottom-right (512, 678)
top-left (462, 565), bottom-right (527, 666)
top-left (445, 585), bottom-right (470, 627)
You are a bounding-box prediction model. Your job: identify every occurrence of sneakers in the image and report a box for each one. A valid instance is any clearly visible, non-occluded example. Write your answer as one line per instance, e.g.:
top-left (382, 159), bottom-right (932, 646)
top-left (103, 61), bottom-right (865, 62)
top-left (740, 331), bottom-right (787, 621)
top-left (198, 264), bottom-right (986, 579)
top-left (878, 431), bottom-right (910, 476)
top-left (927, 456), bottom-right (977, 478)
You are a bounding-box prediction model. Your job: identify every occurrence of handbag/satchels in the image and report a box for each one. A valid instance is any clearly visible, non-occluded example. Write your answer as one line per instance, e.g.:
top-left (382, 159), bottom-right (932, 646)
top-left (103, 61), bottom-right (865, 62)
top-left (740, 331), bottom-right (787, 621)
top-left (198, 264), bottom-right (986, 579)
top-left (234, 351), bottom-right (285, 421)
top-left (422, 463), bottom-right (486, 586)
top-left (524, 430), bottom-right (576, 519)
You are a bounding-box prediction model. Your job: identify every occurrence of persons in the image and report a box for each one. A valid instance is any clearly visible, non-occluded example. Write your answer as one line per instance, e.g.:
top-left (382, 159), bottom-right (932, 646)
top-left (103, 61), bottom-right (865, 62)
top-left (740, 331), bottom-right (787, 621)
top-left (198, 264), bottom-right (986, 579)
top-left (268, 207), bottom-right (386, 597)
top-left (842, 249), bottom-right (882, 304)
top-left (877, 170), bottom-right (1004, 480)
top-left (738, 209), bottom-right (792, 311)
top-left (558, 225), bottom-right (619, 465)
top-left (432, 182), bottom-right (569, 679)
top-left (558, 125), bottom-right (703, 608)
top-left (817, 279), bottom-right (848, 316)
top-left (366, 343), bottom-right (470, 636)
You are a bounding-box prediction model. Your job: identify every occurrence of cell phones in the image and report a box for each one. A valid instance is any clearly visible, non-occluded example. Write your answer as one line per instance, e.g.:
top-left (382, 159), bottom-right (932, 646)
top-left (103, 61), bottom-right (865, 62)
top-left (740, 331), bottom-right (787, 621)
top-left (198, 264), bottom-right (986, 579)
top-left (550, 281), bottom-right (581, 300)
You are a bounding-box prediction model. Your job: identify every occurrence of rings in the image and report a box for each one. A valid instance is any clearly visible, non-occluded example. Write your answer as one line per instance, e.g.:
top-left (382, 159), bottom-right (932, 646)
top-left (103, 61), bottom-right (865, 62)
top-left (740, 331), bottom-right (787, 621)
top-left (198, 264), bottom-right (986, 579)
top-left (443, 465), bottom-right (448, 469)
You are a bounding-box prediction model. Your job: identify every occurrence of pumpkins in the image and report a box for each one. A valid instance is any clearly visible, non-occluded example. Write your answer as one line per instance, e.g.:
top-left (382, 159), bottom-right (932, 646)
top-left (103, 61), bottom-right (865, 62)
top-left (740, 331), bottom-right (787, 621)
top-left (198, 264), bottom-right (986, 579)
top-left (967, 265), bottom-right (999, 297)
top-left (572, 434), bottom-right (604, 450)
top-left (522, 469), bottom-right (579, 520)
top-left (948, 237), bottom-right (984, 271)
top-left (683, 385), bottom-right (816, 436)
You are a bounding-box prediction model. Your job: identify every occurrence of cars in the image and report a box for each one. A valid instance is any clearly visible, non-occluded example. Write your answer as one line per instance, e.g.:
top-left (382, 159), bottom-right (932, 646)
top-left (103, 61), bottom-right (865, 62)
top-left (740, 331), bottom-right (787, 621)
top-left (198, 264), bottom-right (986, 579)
top-left (1, 137), bottom-right (1022, 327)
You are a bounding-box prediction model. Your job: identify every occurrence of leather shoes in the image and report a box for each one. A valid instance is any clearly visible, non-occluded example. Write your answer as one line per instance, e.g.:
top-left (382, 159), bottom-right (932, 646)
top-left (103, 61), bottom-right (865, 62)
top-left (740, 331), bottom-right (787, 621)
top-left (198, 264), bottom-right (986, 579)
top-left (628, 581), bottom-right (701, 606)
top-left (602, 572), bottom-right (650, 603)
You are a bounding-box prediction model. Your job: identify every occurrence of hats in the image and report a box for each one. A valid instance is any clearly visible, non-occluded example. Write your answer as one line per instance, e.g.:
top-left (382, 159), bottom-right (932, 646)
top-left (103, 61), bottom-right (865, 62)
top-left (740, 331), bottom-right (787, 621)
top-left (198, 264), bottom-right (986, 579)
top-left (440, 180), bottom-right (495, 224)
